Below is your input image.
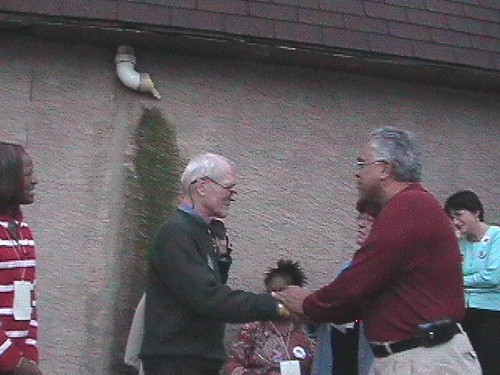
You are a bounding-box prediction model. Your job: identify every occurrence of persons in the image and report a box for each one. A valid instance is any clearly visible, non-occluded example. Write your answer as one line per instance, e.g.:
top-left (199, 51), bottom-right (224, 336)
top-left (445, 188), bottom-right (500, 375)
top-left (142, 151), bottom-right (309, 374)
top-left (1, 141), bottom-right (43, 375)
top-left (271, 124), bottom-right (486, 375)
top-left (224, 258), bottom-right (316, 375)
top-left (305, 196), bottom-right (384, 373)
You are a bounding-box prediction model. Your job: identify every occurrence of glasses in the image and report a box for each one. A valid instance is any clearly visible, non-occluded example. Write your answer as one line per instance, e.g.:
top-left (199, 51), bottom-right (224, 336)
top-left (354, 157), bottom-right (390, 169)
top-left (201, 175), bottom-right (237, 194)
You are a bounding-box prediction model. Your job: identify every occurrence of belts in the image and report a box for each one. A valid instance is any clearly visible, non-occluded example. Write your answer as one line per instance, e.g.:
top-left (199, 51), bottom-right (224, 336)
top-left (369, 325), bottom-right (462, 359)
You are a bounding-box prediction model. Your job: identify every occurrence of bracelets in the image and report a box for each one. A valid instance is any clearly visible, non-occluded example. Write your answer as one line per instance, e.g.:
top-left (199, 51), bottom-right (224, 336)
top-left (18, 356), bottom-right (24, 367)
top-left (276, 299), bottom-right (292, 319)
